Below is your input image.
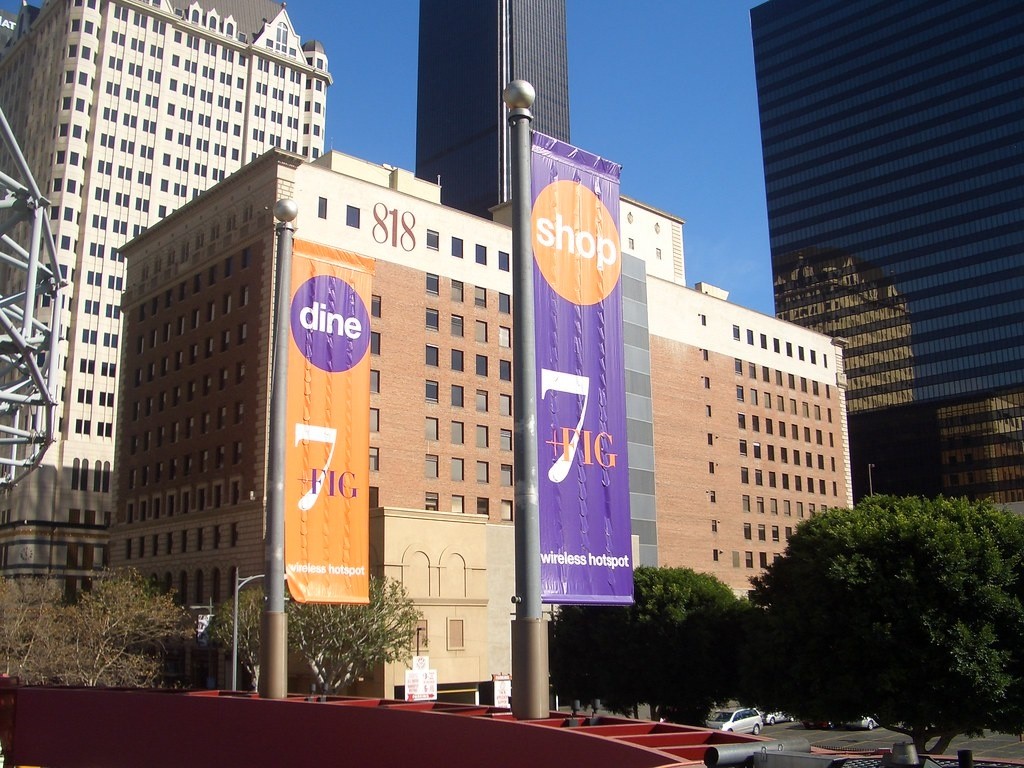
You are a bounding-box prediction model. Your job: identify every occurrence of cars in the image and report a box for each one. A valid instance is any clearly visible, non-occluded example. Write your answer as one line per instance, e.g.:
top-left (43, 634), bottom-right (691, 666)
top-left (705, 706), bottom-right (764, 736)
top-left (841, 714), bottom-right (880, 731)
top-left (801, 719), bottom-right (836, 730)
top-left (763, 711), bottom-right (795, 726)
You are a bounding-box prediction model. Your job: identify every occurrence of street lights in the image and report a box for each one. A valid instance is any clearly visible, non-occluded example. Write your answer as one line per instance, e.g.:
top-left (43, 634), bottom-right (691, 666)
top-left (231, 567), bottom-right (290, 697)
top-left (180, 604), bottom-right (214, 689)
top-left (868, 463), bottom-right (876, 498)
